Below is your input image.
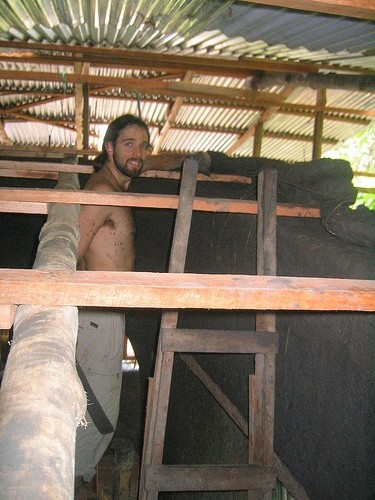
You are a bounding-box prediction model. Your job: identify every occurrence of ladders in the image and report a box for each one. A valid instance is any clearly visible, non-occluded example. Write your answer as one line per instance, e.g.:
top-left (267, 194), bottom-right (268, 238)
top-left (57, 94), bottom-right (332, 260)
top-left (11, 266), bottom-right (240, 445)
top-left (135, 158), bottom-right (281, 500)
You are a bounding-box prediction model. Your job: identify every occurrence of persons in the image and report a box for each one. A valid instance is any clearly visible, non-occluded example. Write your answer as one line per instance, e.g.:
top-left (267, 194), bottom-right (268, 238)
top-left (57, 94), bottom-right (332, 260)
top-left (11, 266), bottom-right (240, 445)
top-left (75, 114), bottom-right (212, 500)
